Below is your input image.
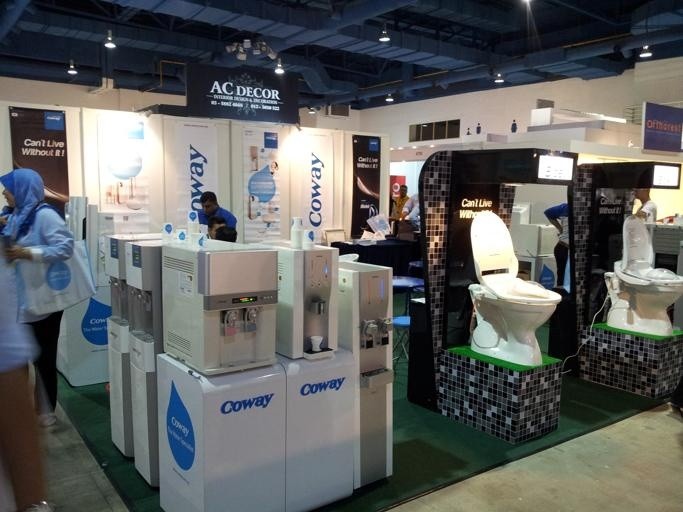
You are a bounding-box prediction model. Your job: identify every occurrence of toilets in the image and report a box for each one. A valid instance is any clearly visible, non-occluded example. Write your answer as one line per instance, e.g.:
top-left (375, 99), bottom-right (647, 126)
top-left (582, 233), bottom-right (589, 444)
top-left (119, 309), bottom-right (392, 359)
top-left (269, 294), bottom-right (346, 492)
top-left (603, 214), bottom-right (683, 337)
top-left (467, 209), bottom-right (564, 367)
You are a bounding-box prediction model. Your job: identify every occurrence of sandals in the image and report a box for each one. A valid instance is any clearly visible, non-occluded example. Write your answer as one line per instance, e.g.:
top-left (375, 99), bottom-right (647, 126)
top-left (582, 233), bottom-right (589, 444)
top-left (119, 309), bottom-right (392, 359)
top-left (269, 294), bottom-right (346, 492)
top-left (670, 403), bottom-right (683, 418)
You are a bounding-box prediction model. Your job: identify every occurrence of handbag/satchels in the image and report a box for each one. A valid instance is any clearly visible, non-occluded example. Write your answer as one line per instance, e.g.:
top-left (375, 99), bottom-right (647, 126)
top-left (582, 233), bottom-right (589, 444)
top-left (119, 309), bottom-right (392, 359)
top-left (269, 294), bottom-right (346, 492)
top-left (11, 208), bottom-right (97, 324)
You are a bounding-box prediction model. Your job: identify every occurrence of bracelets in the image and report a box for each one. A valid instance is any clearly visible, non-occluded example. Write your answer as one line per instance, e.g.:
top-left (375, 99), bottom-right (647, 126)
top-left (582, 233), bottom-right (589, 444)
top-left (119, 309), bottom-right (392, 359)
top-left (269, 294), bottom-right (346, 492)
top-left (22, 500), bottom-right (58, 512)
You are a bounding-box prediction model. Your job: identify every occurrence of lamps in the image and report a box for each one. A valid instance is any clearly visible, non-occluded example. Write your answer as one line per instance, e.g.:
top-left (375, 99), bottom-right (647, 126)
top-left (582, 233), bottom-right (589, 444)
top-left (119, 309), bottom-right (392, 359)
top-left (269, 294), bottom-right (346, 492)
top-left (494, 73), bottom-right (505, 82)
top-left (67, 29), bottom-right (117, 75)
top-left (384, 93), bottom-right (394, 102)
top-left (378, 23), bottom-right (390, 42)
top-left (224, 31), bottom-right (285, 75)
top-left (639, 43), bottom-right (654, 59)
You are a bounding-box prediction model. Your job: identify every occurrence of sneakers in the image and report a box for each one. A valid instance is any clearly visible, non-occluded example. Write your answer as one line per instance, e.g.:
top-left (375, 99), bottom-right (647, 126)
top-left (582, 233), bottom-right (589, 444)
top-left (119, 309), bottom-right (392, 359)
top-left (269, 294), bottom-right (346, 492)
top-left (33, 410), bottom-right (59, 433)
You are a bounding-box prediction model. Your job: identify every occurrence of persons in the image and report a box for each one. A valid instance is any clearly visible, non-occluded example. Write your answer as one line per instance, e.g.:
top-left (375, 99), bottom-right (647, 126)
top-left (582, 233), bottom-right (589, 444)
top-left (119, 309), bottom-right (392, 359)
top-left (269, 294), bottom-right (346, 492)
top-left (1, 233), bottom-right (63, 512)
top-left (1, 167), bottom-right (76, 432)
top-left (207, 215), bottom-right (230, 239)
top-left (396, 185), bottom-right (411, 222)
top-left (215, 226), bottom-right (238, 244)
top-left (634, 187), bottom-right (658, 223)
top-left (542, 203), bottom-right (570, 290)
top-left (200, 192), bottom-right (238, 239)
top-left (400, 193), bottom-right (422, 230)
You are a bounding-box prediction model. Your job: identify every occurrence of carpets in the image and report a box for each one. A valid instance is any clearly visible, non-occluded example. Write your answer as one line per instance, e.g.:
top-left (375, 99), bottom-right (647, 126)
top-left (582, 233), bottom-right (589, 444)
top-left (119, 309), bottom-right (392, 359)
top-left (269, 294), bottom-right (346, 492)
top-left (57, 291), bottom-right (677, 510)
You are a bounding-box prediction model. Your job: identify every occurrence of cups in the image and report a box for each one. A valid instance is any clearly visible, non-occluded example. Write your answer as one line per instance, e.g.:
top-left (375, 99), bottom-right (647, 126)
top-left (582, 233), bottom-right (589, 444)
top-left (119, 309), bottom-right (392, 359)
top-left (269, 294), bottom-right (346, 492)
top-left (311, 336), bottom-right (323, 352)
top-left (291, 217), bottom-right (315, 250)
top-left (162, 210), bottom-right (209, 248)
